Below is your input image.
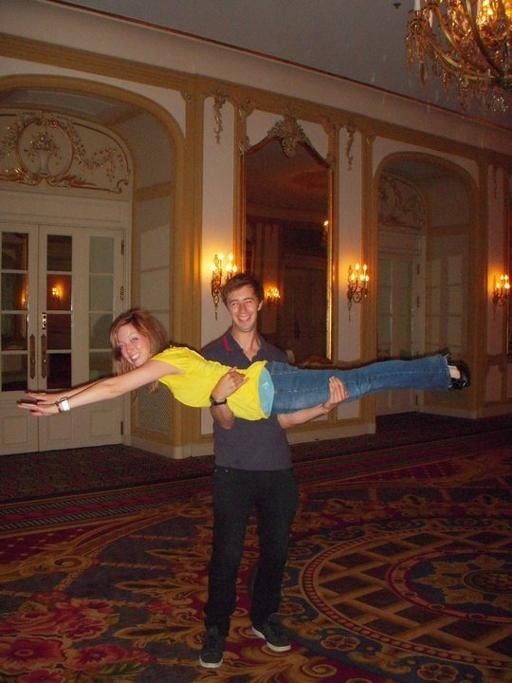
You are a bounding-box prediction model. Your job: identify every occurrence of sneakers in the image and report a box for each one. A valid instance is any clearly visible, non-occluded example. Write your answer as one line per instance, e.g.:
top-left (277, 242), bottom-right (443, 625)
top-left (198, 639), bottom-right (224, 669)
top-left (447, 361), bottom-right (471, 392)
top-left (251, 619), bottom-right (291, 653)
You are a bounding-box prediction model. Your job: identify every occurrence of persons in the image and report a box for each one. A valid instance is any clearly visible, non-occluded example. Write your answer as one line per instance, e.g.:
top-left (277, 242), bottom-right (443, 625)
top-left (200, 273), bottom-right (349, 668)
top-left (15, 308), bottom-right (471, 418)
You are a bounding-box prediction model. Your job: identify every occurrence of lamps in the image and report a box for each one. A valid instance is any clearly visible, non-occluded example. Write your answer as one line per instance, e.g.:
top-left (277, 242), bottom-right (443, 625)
top-left (346, 260), bottom-right (370, 319)
top-left (209, 251), bottom-right (237, 321)
top-left (491, 275), bottom-right (512, 321)
top-left (403, 0), bottom-right (512, 111)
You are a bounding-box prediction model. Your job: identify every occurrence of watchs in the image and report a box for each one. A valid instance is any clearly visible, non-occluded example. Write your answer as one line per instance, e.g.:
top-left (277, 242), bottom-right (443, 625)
top-left (207, 397), bottom-right (226, 405)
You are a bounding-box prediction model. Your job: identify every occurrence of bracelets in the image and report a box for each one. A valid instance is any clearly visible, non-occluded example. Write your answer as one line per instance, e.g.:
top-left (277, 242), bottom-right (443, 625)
top-left (61, 398), bottom-right (70, 412)
top-left (320, 404), bottom-right (329, 414)
top-left (54, 400), bottom-right (62, 415)
